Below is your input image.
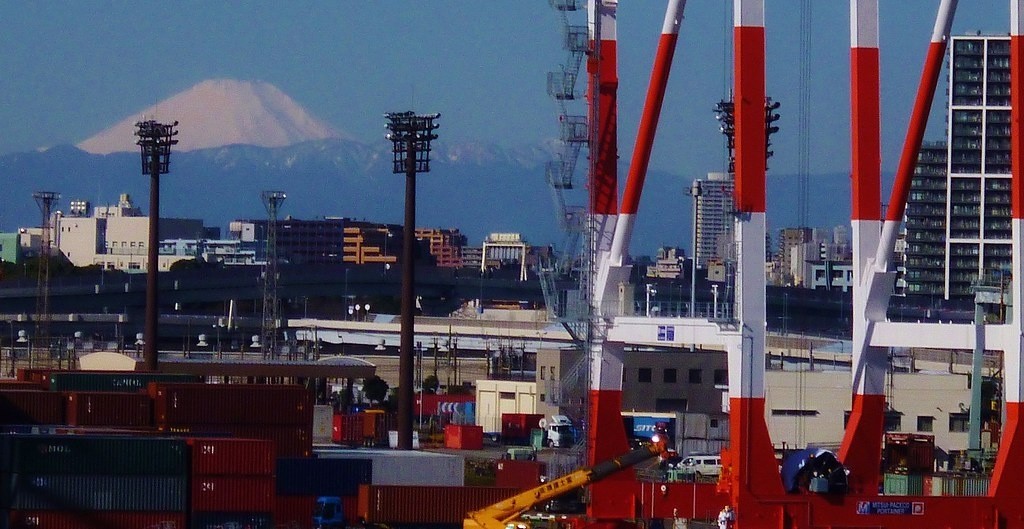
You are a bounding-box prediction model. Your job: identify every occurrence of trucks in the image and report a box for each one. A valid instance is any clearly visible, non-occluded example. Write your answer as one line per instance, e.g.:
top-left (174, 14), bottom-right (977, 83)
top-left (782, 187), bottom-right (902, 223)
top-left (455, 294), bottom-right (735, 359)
top-left (545, 414), bottom-right (573, 449)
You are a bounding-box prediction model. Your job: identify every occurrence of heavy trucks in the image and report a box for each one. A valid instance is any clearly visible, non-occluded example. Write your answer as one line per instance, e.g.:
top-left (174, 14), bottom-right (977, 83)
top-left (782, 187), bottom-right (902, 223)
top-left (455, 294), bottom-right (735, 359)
top-left (313, 484), bottom-right (522, 529)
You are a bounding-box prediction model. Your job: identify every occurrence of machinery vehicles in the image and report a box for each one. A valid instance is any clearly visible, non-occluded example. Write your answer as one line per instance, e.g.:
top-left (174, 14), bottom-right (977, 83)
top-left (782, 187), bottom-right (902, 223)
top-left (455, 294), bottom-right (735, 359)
top-left (464, 433), bottom-right (679, 529)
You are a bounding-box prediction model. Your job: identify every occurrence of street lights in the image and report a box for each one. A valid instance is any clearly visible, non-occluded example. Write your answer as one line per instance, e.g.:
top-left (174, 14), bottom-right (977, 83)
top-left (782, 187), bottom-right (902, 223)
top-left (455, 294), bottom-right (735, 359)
top-left (259, 191), bottom-right (286, 360)
top-left (646, 283), bottom-right (653, 318)
top-left (712, 284), bottom-right (718, 317)
top-left (385, 109), bottom-right (441, 448)
top-left (31, 191), bottom-right (63, 365)
top-left (134, 119), bottom-right (180, 371)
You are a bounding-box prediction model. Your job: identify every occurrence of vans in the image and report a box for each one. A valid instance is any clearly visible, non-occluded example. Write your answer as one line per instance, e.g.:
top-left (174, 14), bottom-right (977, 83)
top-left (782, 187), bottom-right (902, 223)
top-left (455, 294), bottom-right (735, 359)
top-left (676, 455), bottom-right (721, 481)
